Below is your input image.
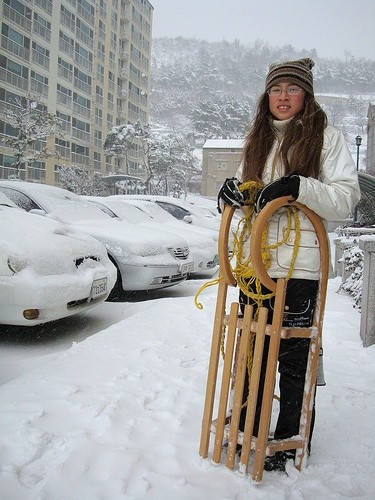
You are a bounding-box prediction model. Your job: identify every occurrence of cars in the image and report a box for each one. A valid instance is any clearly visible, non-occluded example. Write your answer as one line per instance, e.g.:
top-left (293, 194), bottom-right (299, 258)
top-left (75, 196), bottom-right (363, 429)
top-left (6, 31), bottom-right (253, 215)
top-left (1, 180), bottom-right (191, 299)
top-left (83, 195), bottom-right (226, 275)
top-left (1, 190), bottom-right (118, 328)
top-left (176, 192), bottom-right (247, 245)
top-left (109, 194), bottom-right (242, 267)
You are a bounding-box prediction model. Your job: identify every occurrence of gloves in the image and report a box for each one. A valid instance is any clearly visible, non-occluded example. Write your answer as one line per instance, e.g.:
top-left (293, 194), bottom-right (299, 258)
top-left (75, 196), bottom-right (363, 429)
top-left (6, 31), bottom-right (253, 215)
top-left (254, 175), bottom-right (301, 213)
top-left (217, 177), bottom-right (248, 214)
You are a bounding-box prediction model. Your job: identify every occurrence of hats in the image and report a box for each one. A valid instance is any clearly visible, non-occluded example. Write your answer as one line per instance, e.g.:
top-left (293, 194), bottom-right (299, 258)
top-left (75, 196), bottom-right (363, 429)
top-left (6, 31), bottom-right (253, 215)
top-left (266, 58), bottom-right (315, 96)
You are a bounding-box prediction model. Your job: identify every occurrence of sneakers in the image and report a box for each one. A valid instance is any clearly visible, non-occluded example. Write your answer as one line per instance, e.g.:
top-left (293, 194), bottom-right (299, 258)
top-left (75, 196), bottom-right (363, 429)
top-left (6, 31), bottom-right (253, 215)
top-left (264, 451), bottom-right (295, 471)
top-left (224, 442), bottom-right (243, 458)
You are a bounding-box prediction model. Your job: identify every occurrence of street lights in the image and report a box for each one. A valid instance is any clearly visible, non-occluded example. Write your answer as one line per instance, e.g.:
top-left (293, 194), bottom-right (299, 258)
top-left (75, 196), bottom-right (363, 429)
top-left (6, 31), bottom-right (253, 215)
top-left (355, 133), bottom-right (362, 223)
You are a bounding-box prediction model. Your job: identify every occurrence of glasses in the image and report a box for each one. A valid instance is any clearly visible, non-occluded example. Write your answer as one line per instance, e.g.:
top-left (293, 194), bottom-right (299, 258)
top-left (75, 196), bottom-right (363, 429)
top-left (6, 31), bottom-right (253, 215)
top-left (266, 86), bottom-right (304, 95)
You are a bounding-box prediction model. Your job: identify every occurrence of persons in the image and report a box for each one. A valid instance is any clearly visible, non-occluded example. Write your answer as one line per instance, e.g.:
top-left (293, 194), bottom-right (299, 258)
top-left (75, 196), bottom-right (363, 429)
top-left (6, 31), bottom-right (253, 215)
top-left (216, 54), bottom-right (362, 476)
